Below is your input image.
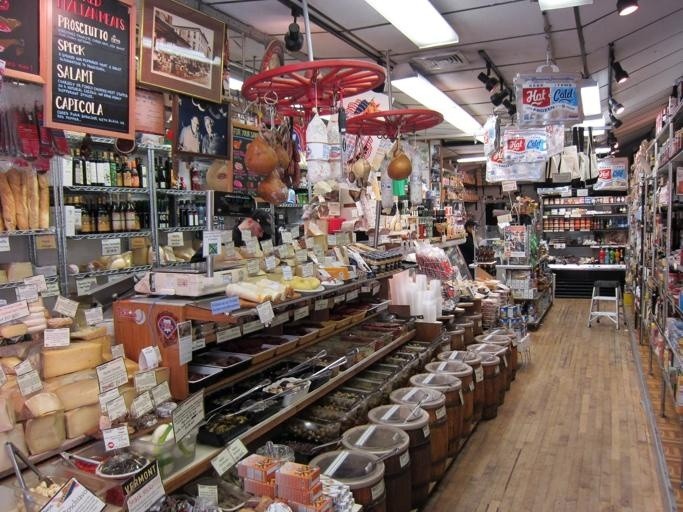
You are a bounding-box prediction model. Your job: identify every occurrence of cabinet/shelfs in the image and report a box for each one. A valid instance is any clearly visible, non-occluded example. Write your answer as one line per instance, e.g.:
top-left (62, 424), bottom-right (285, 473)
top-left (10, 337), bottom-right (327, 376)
top-left (539, 190), bottom-right (628, 299)
top-left (116, 270), bottom-right (446, 495)
top-left (638, 100), bottom-right (683, 418)
top-left (0, 134), bottom-right (214, 290)
top-left (494, 254), bottom-right (556, 332)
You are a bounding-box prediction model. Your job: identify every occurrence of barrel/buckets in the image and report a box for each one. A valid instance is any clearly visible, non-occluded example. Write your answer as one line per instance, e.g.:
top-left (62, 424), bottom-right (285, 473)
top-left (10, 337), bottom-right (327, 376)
top-left (308, 326), bottom-right (518, 512)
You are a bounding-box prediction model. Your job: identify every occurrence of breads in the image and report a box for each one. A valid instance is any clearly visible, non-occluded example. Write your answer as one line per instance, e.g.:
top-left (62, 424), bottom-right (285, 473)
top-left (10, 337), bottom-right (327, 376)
top-left (1, 261), bottom-right (34, 283)
top-left (0, 169), bottom-right (47, 229)
top-left (0, 294), bottom-right (73, 339)
top-left (1, 321), bottom-right (171, 473)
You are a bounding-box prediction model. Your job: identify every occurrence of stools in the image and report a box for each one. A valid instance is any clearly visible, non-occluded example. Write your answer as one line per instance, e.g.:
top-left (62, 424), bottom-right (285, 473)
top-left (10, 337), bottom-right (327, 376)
top-left (586, 280), bottom-right (627, 330)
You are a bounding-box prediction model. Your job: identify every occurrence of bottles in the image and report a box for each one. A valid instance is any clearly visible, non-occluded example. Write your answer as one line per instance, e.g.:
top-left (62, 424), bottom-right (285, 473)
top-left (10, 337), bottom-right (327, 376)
top-left (49, 193), bottom-right (224, 232)
top-left (594, 205), bottom-right (628, 263)
top-left (380, 201), bottom-right (448, 241)
top-left (359, 250), bottom-right (402, 275)
top-left (153, 147), bottom-right (188, 189)
top-left (68, 147), bottom-right (148, 189)
top-left (666, 83), bottom-right (678, 113)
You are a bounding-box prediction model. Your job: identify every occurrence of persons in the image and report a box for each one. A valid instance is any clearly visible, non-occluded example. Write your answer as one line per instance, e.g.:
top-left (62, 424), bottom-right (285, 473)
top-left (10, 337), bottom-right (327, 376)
top-left (201, 115), bottom-right (225, 156)
top-left (177, 113), bottom-right (201, 152)
top-left (607, 230), bottom-right (625, 244)
top-left (188, 207), bottom-right (274, 263)
top-left (459, 219), bottom-right (478, 264)
top-left (518, 204), bottom-right (532, 225)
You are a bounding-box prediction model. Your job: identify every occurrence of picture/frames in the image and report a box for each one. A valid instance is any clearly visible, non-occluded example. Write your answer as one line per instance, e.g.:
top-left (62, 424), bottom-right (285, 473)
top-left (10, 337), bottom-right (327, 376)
top-left (137, 0), bottom-right (226, 107)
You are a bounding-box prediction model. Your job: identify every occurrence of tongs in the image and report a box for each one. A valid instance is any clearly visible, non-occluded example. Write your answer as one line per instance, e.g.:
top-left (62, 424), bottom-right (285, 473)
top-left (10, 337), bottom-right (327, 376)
top-left (5, 438), bottom-right (51, 511)
top-left (58, 448), bottom-right (98, 476)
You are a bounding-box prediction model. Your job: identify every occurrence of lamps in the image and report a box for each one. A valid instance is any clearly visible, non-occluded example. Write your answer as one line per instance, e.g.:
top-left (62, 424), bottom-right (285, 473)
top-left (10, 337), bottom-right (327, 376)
top-left (615, 1), bottom-right (640, 17)
top-left (476, 48), bottom-right (516, 116)
top-left (604, 41), bottom-right (630, 149)
top-left (282, 4), bottom-right (304, 53)
